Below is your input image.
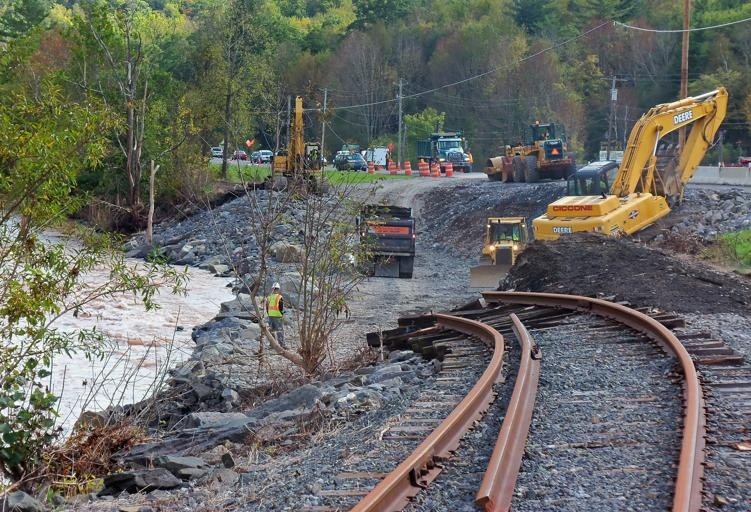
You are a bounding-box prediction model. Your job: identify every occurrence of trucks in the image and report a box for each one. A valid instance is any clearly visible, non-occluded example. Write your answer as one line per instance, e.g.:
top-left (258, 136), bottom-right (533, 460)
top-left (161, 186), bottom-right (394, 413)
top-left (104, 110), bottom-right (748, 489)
top-left (355, 203), bottom-right (416, 278)
top-left (415, 131), bottom-right (471, 172)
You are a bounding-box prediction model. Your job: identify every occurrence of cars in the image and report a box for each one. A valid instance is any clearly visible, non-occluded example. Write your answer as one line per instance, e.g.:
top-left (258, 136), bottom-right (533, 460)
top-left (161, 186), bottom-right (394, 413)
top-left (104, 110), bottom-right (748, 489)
top-left (250, 150), bottom-right (273, 163)
top-left (334, 152), bottom-right (368, 171)
top-left (232, 151), bottom-right (248, 161)
top-left (210, 146), bottom-right (223, 157)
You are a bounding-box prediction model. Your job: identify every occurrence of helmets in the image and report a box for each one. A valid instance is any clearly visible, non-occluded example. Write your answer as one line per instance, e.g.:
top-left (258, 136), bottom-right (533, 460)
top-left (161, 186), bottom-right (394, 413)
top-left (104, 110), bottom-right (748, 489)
top-left (271, 282), bottom-right (280, 289)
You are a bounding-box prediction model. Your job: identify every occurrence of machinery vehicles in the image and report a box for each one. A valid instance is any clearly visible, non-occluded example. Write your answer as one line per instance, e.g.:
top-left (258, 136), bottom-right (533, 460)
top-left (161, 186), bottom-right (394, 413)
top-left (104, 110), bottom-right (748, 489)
top-left (531, 87), bottom-right (730, 240)
top-left (470, 216), bottom-right (531, 288)
top-left (482, 119), bottom-right (576, 182)
top-left (269, 96), bottom-right (329, 194)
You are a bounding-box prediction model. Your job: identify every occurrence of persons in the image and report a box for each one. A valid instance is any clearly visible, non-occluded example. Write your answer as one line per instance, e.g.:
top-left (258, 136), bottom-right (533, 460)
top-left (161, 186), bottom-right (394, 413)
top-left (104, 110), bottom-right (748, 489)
top-left (385, 151), bottom-right (393, 170)
top-left (265, 282), bottom-right (288, 351)
top-left (500, 226), bottom-right (519, 241)
top-left (586, 173), bottom-right (608, 194)
